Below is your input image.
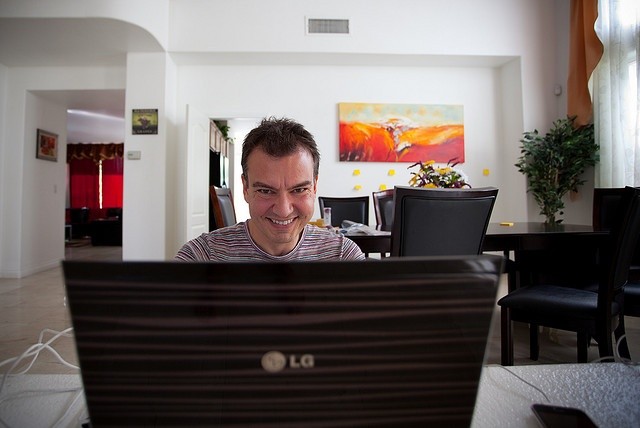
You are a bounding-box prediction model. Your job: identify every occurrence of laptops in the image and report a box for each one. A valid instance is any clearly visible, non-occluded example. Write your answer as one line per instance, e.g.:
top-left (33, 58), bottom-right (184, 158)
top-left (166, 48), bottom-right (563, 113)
top-left (58, 255), bottom-right (501, 426)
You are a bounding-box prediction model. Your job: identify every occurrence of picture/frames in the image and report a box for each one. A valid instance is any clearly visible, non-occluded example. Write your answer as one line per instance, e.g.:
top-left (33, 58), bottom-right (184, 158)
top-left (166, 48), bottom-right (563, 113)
top-left (37, 127), bottom-right (60, 162)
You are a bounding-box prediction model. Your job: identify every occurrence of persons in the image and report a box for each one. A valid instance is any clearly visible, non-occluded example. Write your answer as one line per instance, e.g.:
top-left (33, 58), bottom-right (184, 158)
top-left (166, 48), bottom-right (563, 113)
top-left (171, 117), bottom-right (368, 260)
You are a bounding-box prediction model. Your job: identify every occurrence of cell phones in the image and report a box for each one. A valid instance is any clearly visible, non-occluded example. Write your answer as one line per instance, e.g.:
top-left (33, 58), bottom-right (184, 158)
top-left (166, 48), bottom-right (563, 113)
top-left (530, 401), bottom-right (596, 428)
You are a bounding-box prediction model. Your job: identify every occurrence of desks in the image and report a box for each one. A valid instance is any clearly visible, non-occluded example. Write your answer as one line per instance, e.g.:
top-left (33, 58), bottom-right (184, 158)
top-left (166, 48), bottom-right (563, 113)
top-left (334, 222), bottom-right (591, 252)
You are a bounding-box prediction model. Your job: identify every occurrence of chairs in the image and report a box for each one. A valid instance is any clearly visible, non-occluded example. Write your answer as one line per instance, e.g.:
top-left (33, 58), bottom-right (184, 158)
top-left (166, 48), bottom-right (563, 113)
top-left (371, 186), bottom-right (414, 257)
top-left (389, 189), bottom-right (498, 257)
top-left (593, 188), bottom-right (639, 318)
top-left (496, 186), bottom-right (640, 364)
top-left (207, 183), bottom-right (239, 230)
top-left (318, 194), bottom-right (370, 260)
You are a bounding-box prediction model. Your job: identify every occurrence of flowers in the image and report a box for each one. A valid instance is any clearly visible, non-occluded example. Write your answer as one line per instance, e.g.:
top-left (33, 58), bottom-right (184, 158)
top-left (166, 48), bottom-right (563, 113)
top-left (405, 157), bottom-right (475, 190)
